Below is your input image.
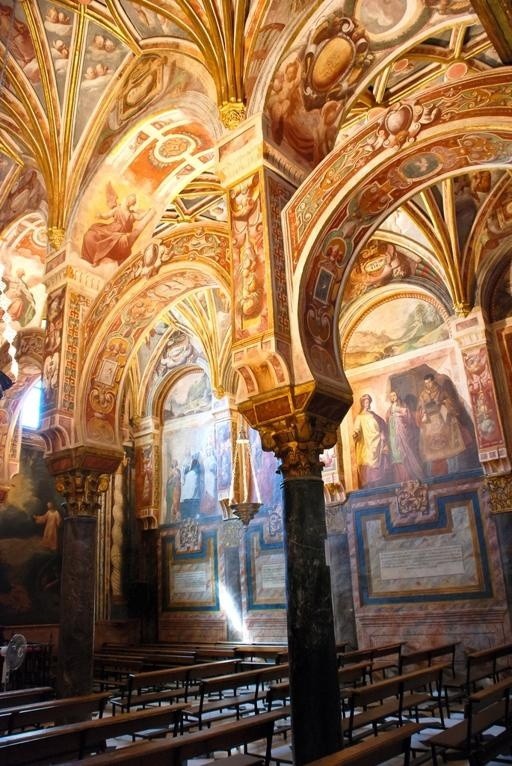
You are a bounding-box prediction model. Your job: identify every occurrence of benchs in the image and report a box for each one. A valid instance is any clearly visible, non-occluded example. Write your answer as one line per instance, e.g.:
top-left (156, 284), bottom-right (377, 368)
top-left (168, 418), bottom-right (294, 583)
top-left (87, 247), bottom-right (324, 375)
top-left (1, 635), bottom-right (510, 760)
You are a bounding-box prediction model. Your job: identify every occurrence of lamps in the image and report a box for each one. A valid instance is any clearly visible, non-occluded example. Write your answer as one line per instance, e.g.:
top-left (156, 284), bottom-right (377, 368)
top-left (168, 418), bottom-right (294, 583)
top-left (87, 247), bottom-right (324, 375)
top-left (222, 413), bottom-right (263, 524)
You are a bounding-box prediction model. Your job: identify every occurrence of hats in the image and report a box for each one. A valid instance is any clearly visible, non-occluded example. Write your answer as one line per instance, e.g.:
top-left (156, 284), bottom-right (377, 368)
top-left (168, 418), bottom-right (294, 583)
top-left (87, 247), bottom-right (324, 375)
top-left (360, 394), bottom-right (372, 402)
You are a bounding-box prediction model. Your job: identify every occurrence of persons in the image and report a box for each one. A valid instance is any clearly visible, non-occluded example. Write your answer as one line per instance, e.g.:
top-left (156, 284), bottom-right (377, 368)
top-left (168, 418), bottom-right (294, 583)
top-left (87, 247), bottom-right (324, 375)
top-left (33, 502), bottom-right (62, 552)
top-left (386, 391), bottom-right (428, 483)
top-left (81, 192), bottom-right (151, 267)
top-left (167, 446), bottom-right (217, 522)
top-left (416, 374), bottom-right (474, 476)
top-left (351, 394), bottom-right (394, 489)
